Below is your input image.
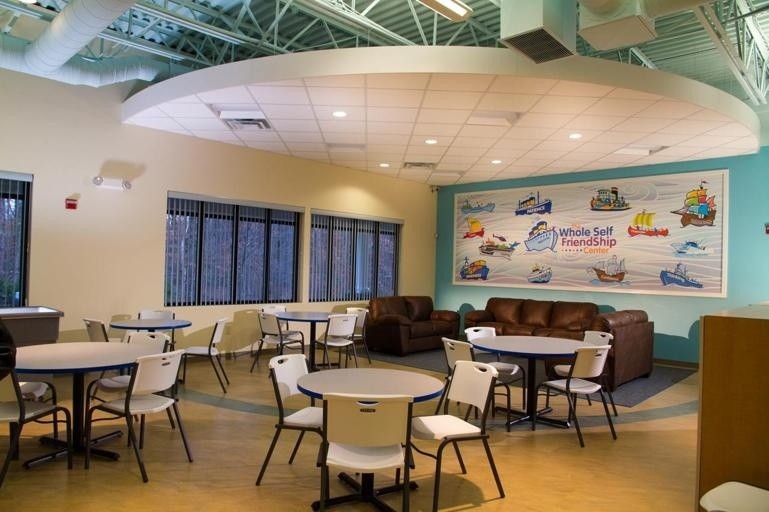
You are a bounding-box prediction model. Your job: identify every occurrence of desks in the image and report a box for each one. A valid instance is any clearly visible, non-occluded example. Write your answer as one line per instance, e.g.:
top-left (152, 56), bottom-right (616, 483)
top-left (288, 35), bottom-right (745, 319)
top-left (298, 368), bottom-right (445, 511)
top-left (272, 311), bottom-right (340, 374)
top-left (110, 318), bottom-right (190, 354)
top-left (469, 335), bottom-right (593, 430)
top-left (10, 340), bottom-right (163, 468)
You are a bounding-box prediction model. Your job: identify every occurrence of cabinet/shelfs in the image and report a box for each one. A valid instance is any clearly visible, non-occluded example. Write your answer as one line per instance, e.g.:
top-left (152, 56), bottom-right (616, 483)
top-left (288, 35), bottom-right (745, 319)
top-left (696, 303), bottom-right (769, 512)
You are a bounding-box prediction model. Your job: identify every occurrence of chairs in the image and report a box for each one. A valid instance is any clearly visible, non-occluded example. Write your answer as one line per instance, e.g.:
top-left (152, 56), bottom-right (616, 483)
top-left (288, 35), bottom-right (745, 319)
top-left (399, 360), bottom-right (505, 512)
top-left (339, 308), bottom-right (371, 367)
top-left (84, 319), bottom-right (107, 342)
top-left (545, 331), bottom-right (618, 418)
top-left (257, 307), bottom-right (305, 356)
top-left (532, 344), bottom-right (617, 446)
top-left (0, 324), bottom-right (72, 490)
top-left (436, 337), bottom-right (511, 434)
top-left (466, 327), bottom-right (526, 412)
top-left (313, 394), bottom-right (414, 512)
top-left (85, 351), bottom-right (193, 482)
top-left (700, 478), bottom-right (769, 512)
top-left (137, 311), bottom-right (175, 353)
top-left (250, 313), bottom-right (304, 373)
top-left (86, 334), bottom-right (174, 447)
top-left (315, 315), bottom-right (359, 370)
top-left (256, 355), bottom-right (332, 485)
top-left (172, 319), bottom-right (229, 393)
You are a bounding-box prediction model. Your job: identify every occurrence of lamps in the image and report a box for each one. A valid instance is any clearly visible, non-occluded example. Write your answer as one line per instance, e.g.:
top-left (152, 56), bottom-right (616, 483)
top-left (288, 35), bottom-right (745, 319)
top-left (452, 170), bottom-right (730, 298)
top-left (417, 0), bottom-right (472, 23)
top-left (93, 176), bottom-right (131, 191)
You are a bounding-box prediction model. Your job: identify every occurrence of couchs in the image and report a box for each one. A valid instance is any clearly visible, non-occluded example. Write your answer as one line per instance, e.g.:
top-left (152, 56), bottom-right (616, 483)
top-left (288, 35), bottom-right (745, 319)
top-left (365, 295), bottom-right (459, 355)
top-left (465, 297), bottom-right (654, 392)
